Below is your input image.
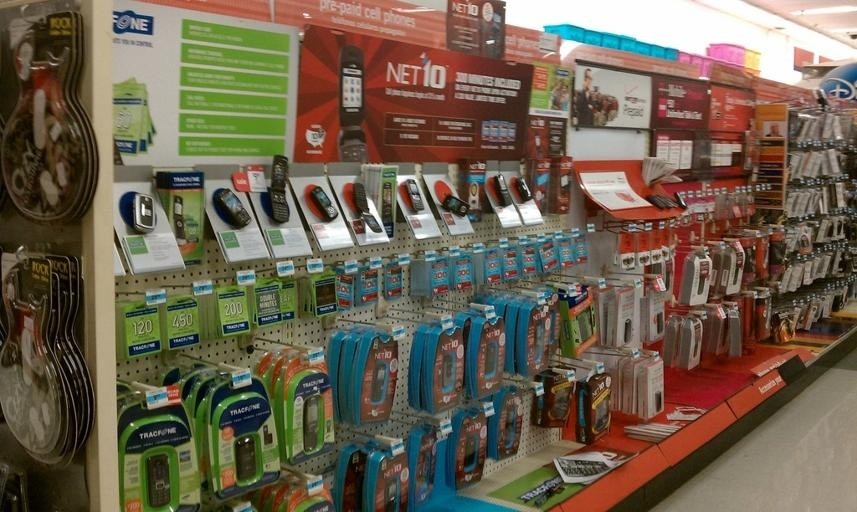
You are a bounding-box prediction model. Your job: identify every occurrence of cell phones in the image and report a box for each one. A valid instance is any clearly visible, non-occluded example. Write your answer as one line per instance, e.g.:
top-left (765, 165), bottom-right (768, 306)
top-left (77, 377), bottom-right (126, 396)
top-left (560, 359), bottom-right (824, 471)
top-left (486, 341), bottom-right (495, 372)
top-left (493, 172), bottom-right (513, 208)
top-left (443, 355), bottom-right (452, 387)
top-left (352, 182), bottom-right (383, 233)
top-left (560, 174), bottom-right (570, 197)
top-left (305, 397), bottom-right (320, 450)
top-left (533, 132), bottom-right (544, 160)
top-left (236, 437), bottom-right (257, 480)
top-left (372, 363), bottom-right (387, 403)
top-left (215, 189), bottom-right (253, 230)
top-left (441, 193), bottom-right (471, 217)
top-left (338, 45), bottom-right (367, 162)
top-left (465, 436), bottom-right (476, 466)
top-left (384, 479), bottom-right (398, 512)
top-left (647, 192), bottom-right (688, 209)
top-left (405, 179), bottom-right (425, 213)
top-left (133, 193), bottom-right (156, 232)
top-left (146, 452), bottom-right (171, 508)
top-left (311, 186), bottom-right (339, 222)
top-left (516, 176), bottom-right (532, 201)
top-left (266, 155), bottom-right (290, 223)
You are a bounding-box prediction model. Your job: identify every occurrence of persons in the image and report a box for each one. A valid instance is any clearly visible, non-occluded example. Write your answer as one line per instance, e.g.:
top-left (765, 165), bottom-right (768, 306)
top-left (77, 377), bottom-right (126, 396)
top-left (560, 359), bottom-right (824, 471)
top-left (764, 122), bottom-right (784, 138)
top-left (576, 68), bottom-right (601, 126)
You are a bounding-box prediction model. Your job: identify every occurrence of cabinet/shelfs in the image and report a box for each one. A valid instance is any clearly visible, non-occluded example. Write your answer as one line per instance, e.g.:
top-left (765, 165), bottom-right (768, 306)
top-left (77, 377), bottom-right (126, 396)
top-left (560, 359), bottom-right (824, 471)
top-left (0, 1), bottom-right (857, 512)
